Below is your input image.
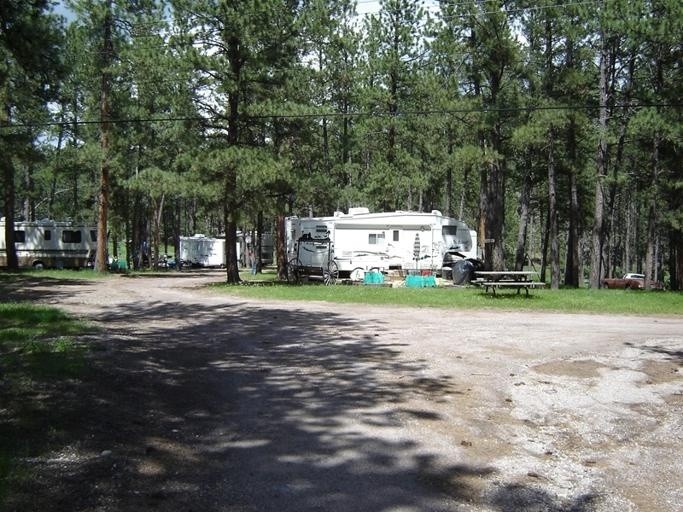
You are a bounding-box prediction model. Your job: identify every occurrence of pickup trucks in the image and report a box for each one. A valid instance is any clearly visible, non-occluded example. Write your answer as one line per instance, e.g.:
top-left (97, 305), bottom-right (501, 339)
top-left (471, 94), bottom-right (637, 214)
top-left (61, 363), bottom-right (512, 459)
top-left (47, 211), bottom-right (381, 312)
top-left (603, 273), bottom-right (666, 292)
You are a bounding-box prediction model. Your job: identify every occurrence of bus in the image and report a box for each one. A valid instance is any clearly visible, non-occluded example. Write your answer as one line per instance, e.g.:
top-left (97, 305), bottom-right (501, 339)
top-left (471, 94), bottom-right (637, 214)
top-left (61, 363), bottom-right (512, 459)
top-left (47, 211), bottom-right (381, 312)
top-left (0, 219), bottom-right (108, 269)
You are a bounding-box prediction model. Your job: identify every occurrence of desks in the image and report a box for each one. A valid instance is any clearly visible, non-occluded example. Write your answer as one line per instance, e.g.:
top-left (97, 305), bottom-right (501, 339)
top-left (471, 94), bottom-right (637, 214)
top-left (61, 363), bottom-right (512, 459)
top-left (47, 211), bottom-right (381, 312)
top-left (474, 269), bottom-right (539, 297)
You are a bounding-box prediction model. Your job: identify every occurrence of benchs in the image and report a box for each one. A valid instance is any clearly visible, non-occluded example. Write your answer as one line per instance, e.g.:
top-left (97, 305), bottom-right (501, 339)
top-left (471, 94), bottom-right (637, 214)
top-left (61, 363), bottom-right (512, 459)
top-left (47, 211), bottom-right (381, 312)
top-left (467, 278), bottom-right (546, 297)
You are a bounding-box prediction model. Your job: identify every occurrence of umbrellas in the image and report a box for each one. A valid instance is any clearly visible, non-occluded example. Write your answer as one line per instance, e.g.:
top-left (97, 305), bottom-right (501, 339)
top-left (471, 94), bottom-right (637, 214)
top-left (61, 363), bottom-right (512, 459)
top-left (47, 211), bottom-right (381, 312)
top-left (411, 233), bottom-right (420, 276)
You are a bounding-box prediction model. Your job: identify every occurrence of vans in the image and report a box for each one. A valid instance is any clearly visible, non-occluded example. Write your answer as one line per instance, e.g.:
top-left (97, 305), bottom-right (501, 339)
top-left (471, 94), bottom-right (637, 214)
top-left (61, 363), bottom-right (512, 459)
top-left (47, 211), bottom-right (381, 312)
top-left (239, 233), bottom-right (274, 267)
